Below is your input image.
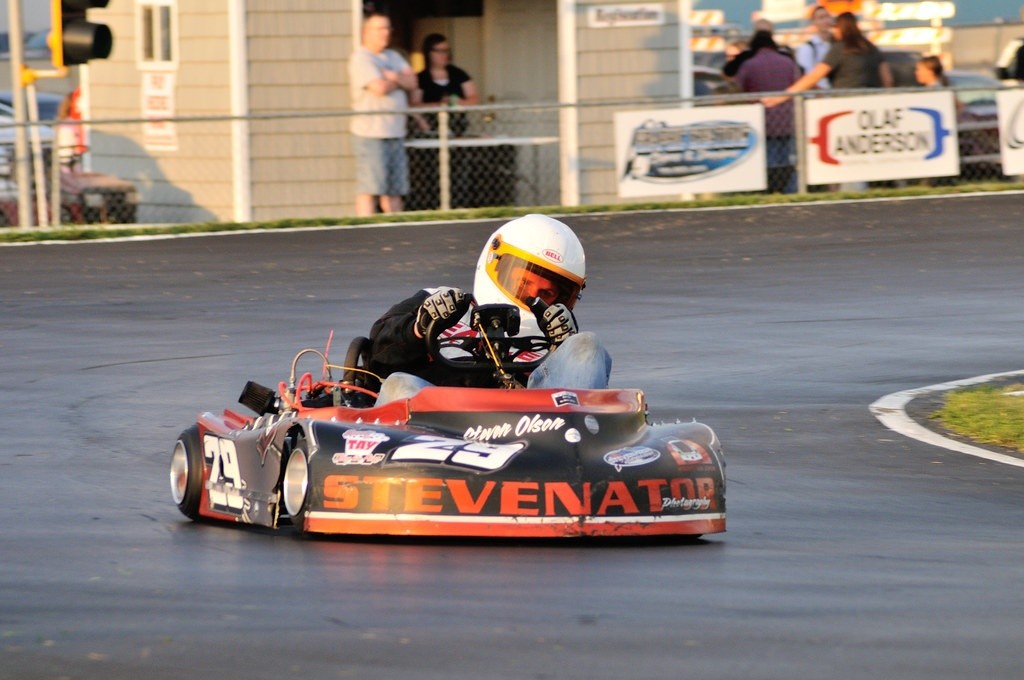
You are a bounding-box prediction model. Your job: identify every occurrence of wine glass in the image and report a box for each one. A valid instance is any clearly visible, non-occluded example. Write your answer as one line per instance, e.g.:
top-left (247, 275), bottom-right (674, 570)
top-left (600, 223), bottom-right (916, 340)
top-left (479, 110), bottom-right (496, 139)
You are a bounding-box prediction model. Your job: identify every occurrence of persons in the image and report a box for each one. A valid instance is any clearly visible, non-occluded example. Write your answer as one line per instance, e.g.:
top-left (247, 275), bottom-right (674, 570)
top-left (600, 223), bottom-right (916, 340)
top-left (914, 56), bottom-right (958, 187)
top-left (413, 31), bottom-right (480, 137)
top-left (349, 15), bottom-right (419, 216)
top-left (758, 12), bottom-right (895, 189)
top-left (721, 18), bottom-right (804, 81)
top-left (369, 213), bottom-right (613, 407)
top-left (733, 30), bottom-right (803, 194)
top-left (56, 92), bottom-right (81, 172)
top-left (796, 6), bottom-right (841, 89)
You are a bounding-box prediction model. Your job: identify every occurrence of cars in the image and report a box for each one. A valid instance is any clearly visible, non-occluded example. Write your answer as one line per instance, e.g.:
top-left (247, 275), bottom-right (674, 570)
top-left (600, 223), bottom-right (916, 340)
top-left (944, 70), bottom-right (1018, 183)
top-left (0, 89), bottom-right (138, 234)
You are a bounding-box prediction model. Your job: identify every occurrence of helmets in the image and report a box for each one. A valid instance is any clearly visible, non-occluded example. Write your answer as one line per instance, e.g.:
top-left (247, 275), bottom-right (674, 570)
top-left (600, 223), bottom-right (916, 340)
top-left (474, 213), bottom-right (586, 346)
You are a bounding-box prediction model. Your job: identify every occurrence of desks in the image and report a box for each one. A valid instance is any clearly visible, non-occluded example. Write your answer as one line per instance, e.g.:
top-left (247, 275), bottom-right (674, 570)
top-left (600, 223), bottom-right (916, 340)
top-left (61, 172), bottom-right (135, 225)
top-left (404, 136), bottom-right (557, 206)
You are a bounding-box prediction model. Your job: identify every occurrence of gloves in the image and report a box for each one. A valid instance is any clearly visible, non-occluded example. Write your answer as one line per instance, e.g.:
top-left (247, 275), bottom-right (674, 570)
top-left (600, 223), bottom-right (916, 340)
top-left (417, 287), bottom-right (473, 339)
top-left (526, 296), bottom-right (580, 347)
